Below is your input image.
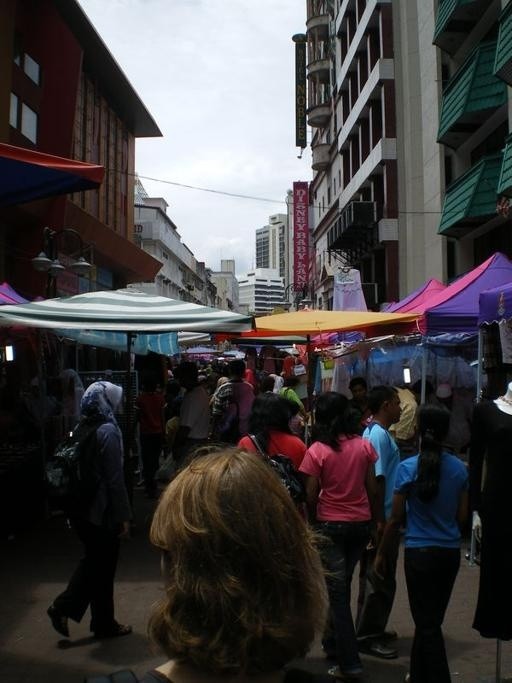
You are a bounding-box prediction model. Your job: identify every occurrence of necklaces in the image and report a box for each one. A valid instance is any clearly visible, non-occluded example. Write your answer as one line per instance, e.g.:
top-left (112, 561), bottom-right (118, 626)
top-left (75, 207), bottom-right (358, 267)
top-left (499, 396), bottom-right (512, 405)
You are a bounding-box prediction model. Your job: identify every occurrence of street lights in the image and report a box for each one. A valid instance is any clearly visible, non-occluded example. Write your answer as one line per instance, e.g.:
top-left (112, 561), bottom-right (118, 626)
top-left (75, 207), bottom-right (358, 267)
top-left (29, 221), bottom-right (92, 298)
top-left (281, 281), bottom-right (312, 312)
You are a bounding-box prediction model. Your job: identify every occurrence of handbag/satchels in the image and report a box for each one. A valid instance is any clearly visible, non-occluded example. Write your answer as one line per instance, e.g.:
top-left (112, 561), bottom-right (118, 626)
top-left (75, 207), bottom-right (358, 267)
top-left (264, 454), bottom-right (306, 504)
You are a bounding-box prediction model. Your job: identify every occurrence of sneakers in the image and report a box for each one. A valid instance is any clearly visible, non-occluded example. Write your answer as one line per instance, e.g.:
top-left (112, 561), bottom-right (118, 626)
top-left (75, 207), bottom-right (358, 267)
top-left (343, 666), bottom-right (365, 677)
top-left (46, 605), bottom-right (70, 637)
top-left (95, 624), bottom-right (132, 639)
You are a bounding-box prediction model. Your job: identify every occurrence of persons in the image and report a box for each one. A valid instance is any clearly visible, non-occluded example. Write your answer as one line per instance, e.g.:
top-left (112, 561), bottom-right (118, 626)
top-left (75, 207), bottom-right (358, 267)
top-left (46, 379), bottom-right (133, 639)
top-left (90, 355), bottom-right (469, 682)
top-left (470, 382), bottom-right (512, 682)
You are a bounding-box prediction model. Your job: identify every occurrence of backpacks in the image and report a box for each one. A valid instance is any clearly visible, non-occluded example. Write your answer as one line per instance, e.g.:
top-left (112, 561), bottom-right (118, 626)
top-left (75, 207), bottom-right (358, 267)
top-left (40, 420), bottom-right (107, 508)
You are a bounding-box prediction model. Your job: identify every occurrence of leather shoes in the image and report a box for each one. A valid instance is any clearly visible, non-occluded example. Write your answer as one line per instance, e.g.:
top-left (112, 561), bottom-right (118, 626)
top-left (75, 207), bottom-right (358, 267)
top-left (383, 630), bottom-right (399, 639)
top-left (356, 640), bottom-right (398, 658)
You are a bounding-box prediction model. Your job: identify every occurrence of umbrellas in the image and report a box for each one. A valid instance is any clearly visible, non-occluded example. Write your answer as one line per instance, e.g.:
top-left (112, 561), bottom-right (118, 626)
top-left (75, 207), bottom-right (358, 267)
top-left (215, 301), bottom-right (424, 341)
top-left (0, 286), bottom-right (256, 371)
